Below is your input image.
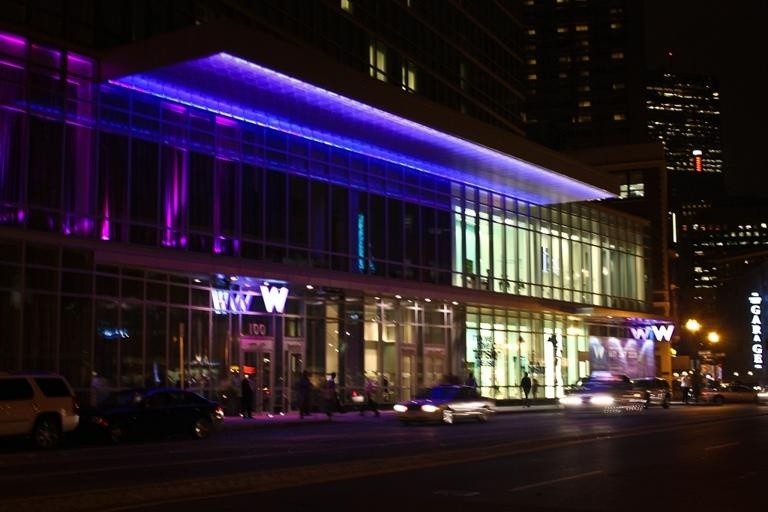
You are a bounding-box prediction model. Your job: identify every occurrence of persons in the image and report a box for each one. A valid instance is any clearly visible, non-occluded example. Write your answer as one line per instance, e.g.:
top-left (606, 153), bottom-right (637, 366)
top-left (520, 371), bottom-right (531, 399)
top-left (240, 375), bottom-right (254, 420)
top-left (466, 372), bottom-right (476, 394)
top-left (327, 371), bottom-right (345, 417)
top-left (297, 370), bottom-right (313, 420)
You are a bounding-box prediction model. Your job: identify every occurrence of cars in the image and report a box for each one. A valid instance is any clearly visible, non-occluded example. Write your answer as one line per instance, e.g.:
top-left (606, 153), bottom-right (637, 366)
top-left (92, 386), bottom-right (224, 445)
top-left (633, 377), bottom-right (671, 409)
top-left (394, 384), bottom-right (495, 424)
top-left (701, 385), bottom-right (759, 403)
top-left (562, 376), bottom-right (641, 416)
top-left (0, 372), bottom-right (79, 450)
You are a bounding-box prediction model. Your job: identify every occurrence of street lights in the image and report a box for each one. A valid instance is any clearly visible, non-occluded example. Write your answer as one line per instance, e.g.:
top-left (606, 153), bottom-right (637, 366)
top-left (685, 311), bottom-right (700, 369)
top-left (709, 329), bottom-right (717, 380)
